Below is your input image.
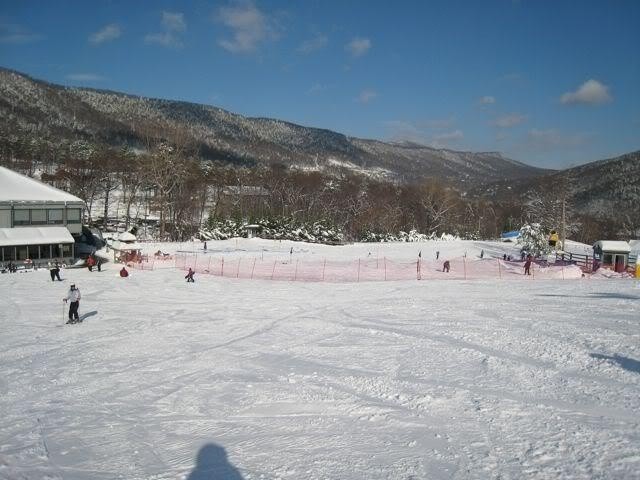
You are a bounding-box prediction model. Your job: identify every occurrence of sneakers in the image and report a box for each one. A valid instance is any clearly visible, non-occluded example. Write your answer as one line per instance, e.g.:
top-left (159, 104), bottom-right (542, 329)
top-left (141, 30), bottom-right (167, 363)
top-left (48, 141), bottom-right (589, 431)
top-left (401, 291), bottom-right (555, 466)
top-left (67, 319), bottom-right (79, 323)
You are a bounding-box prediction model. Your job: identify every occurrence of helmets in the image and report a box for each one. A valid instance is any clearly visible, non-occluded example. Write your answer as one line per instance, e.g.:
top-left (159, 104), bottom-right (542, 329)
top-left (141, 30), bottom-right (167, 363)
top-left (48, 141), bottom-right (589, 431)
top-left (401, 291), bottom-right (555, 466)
top-left (71, 283), bottom-right (75, 287)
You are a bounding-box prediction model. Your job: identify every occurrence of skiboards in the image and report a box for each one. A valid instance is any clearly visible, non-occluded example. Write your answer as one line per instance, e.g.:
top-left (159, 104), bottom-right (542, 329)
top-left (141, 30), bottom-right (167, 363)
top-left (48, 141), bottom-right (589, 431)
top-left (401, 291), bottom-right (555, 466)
top-left (56, 319), bottom-right (83, 328)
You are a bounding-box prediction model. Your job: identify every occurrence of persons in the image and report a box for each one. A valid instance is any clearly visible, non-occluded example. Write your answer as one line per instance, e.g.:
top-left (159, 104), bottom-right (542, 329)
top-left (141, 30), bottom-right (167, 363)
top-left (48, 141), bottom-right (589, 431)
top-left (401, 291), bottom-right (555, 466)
top-left (442, 260), bottom-right (450, 272)
top-left (503, 252), bottom-right (532, 275)
top-left (63, 280), bottom-right (81, 325)
top-left (120, 266), bottom-right (128, 277)
top-left (96, 260), bottom-right (102, 271)
top-left (87, 254), bottom-right (94, 272)
top-left (481, 249), bottom-right (484, 258)
top-left (49, 259), bottom-right (61, 282)
top-left (186, 268), bottom-right (194, 282)
top-left (419, 251), bottom-right (421, 257)
top-left (436, 251), bottom-right (439, 258)
top-left (203, 242), bottom-right (207, 252)
